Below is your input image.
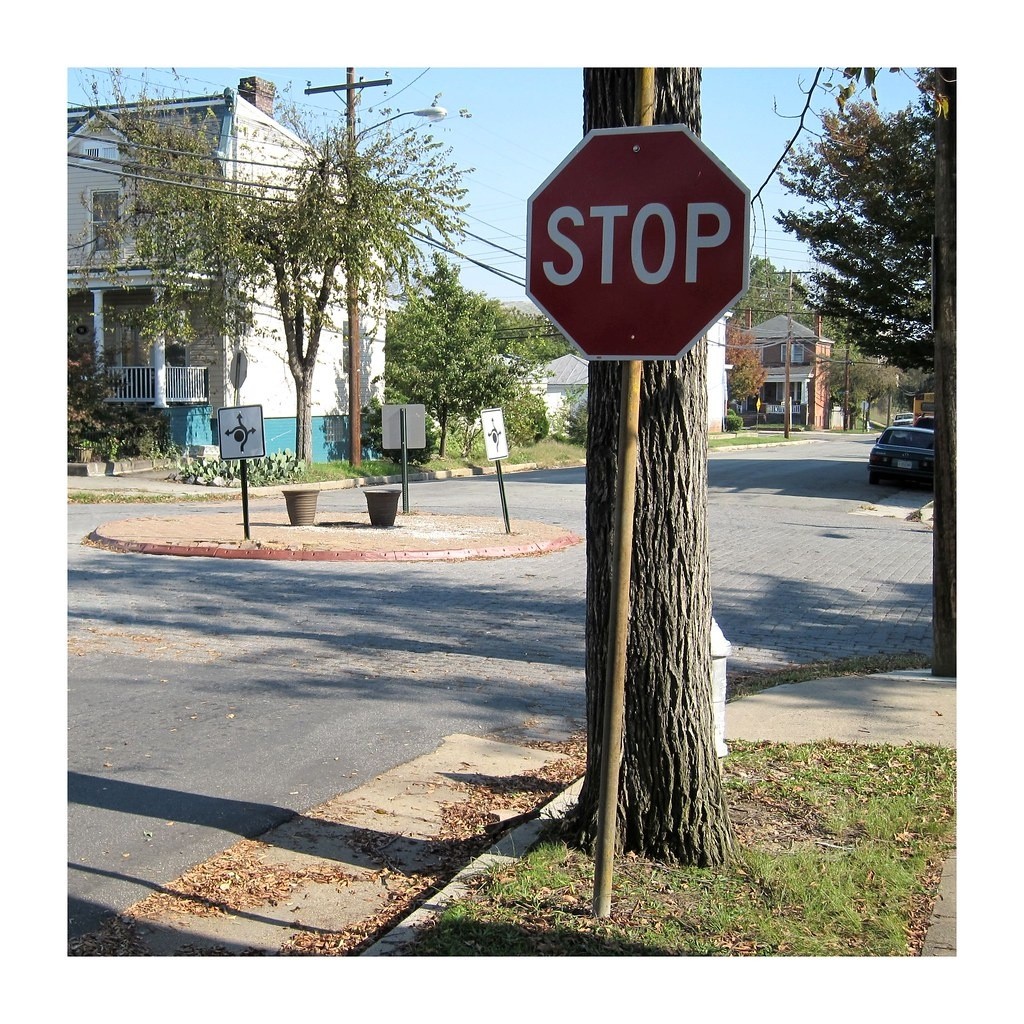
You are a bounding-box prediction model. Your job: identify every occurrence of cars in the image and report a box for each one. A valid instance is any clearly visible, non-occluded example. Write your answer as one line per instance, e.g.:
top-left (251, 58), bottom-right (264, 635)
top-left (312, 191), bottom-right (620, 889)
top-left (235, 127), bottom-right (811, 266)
top-left (868, 426), bottom-right (936, 486)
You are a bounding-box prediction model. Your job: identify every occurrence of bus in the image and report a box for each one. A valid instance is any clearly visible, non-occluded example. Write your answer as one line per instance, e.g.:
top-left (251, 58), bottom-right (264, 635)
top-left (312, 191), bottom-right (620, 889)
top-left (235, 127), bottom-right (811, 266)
top-left (913, 393), bottom-right (935, 419)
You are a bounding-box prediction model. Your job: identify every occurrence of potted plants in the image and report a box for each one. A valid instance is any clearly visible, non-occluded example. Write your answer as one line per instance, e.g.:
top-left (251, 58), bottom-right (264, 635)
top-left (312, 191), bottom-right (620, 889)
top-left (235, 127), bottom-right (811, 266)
top-left (74, 439), bottom-right (95, 463)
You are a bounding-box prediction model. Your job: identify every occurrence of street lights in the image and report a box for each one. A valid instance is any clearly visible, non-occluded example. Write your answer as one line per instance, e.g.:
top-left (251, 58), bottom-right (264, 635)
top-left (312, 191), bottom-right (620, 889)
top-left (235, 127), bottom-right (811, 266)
top-left (343, 107), bottom-right (449, 470)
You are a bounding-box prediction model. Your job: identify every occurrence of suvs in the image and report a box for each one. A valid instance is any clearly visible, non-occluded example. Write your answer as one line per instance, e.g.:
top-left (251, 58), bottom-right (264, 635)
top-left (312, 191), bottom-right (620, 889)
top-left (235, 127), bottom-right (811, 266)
top-left (893, 413), bottom-right (914, 427)
top-left (914, 413), bottom-right (936, 431)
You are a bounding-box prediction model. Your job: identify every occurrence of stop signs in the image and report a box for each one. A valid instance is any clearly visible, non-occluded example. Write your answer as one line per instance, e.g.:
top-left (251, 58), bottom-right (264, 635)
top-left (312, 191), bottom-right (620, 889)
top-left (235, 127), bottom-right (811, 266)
top-left (523, 126), bottom-right (753, 360)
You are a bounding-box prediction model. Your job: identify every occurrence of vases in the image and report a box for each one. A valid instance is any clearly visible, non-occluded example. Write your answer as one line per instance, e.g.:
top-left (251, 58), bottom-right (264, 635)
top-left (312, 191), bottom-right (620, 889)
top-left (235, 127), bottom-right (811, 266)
top-left (282, 489), bottom-right (320, 524)
top-left (362, 488), bottom-right (403, 527)
